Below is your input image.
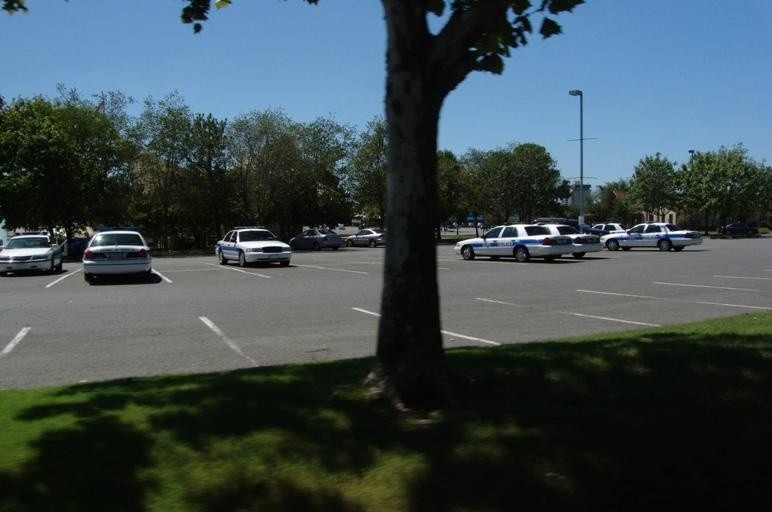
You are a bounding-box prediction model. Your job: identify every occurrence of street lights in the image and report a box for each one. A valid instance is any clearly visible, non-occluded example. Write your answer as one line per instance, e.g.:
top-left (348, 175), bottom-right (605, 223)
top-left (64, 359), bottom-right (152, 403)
top-left (569, 89), bottom-right (584, 227)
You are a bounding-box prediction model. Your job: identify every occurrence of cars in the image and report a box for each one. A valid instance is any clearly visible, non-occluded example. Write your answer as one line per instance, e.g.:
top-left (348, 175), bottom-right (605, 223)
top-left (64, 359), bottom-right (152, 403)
top-left (454, 217), bottom-right (705, 263)
top-left (82, 231), bottom-right (150, 280)
top-left (214, 228), bottom-right (292, 267)
top-left (344, 227), bottom-right (386, 247)
top-left (0, 232), bottom-right (63, 275)
top-left (289, 230), bottom-right (341, 252)
